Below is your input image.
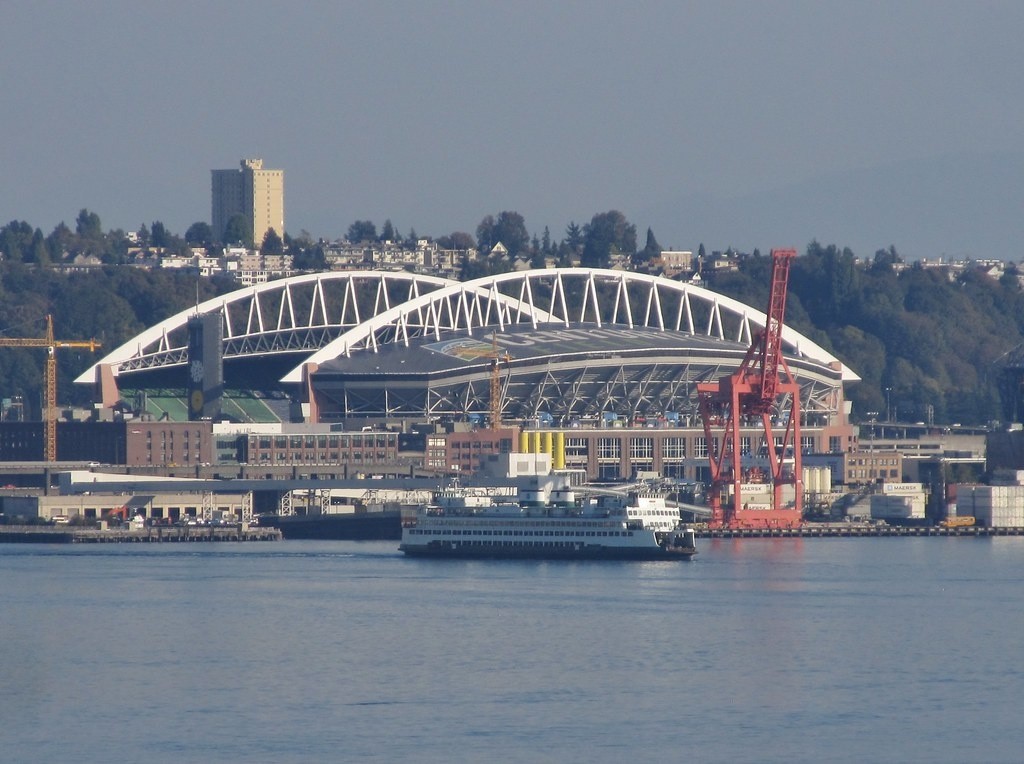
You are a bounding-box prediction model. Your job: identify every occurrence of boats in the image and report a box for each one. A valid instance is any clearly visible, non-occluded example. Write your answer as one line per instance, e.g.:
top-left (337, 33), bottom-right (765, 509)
top-left (398, 474), bottom-right (699, 559)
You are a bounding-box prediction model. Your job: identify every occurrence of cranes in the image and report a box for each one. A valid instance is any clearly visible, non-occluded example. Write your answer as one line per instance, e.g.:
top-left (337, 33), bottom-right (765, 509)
top-left (0, 314), bottom-right (102, 462)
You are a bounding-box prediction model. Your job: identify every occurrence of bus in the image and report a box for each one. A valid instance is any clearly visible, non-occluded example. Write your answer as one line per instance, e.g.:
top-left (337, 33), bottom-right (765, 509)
top-left (939, 516), bottom-right (976, 529)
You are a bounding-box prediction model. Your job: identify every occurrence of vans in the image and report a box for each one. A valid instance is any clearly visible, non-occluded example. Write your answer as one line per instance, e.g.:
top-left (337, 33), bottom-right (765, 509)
top-left (51, 516), bottom-right (66, 523)
